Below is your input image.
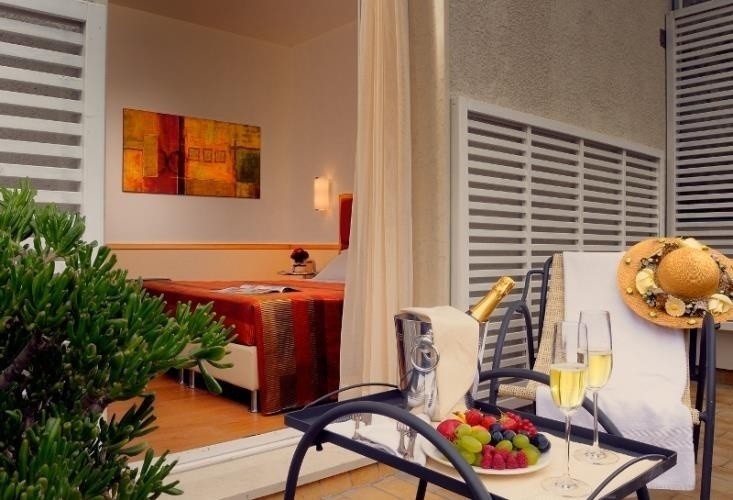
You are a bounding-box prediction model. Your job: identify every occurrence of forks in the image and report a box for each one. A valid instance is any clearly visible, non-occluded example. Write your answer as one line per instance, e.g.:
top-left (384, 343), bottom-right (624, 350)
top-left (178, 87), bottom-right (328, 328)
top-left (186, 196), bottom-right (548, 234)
top-left (396, 419), bottom-right (409, 458)
top-left (405, 426), bottom-right (418, 460)
top-left (351, 411), bottom-right (363, 441)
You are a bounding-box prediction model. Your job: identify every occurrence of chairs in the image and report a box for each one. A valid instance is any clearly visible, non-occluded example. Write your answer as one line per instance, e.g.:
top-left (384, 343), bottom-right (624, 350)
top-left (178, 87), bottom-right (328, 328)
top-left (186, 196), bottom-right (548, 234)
top-left (485, 247), bottom-right (720, 499)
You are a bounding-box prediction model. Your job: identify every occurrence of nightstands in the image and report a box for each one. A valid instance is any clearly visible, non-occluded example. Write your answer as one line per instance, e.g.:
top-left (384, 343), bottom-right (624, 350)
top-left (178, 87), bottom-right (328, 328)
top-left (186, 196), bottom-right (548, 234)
top-left (276, 271), bottom-right (318, 279)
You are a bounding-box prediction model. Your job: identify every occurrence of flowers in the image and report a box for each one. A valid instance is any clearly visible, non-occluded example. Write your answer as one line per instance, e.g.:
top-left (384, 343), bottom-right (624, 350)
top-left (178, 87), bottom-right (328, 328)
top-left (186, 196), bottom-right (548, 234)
top-left (290, 248), bottom-right (309, 264)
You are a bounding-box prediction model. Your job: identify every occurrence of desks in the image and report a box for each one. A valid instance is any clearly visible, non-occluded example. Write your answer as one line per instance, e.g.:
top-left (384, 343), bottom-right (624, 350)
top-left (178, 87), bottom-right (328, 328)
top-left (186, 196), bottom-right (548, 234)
top-left (280, 366), bottom-right (678, 500)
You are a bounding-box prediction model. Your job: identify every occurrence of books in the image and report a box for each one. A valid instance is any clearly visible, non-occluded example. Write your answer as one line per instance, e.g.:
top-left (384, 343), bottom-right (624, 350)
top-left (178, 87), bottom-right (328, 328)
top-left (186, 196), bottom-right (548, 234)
top-left (210, 281), bottom-right (303, 297)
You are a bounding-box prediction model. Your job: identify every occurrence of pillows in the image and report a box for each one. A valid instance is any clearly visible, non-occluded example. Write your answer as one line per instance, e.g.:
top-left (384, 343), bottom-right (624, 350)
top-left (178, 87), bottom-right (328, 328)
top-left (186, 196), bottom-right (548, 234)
top-left (310, 249), bottom-right (347, 283)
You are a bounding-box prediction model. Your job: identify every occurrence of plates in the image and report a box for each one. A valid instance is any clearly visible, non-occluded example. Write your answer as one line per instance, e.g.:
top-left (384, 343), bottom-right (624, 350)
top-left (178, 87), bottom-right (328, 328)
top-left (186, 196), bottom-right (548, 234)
top-left (420, 423), bottom-right (554, 478)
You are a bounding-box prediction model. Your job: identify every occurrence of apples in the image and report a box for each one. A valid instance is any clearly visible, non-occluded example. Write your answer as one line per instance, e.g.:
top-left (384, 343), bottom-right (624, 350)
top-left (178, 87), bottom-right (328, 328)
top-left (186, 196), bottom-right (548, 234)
top-left (435, 419), bottom-right (462, 439)
top-left (465, 409), bottom-right (483, 425)
top-left (481, 415), bottom-right (496, 429)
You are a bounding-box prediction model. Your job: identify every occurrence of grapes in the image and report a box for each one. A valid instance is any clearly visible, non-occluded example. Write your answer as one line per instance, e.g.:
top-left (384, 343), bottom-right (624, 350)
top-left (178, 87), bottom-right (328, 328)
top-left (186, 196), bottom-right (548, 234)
top-left (445, 422), bottom-right (551, 466)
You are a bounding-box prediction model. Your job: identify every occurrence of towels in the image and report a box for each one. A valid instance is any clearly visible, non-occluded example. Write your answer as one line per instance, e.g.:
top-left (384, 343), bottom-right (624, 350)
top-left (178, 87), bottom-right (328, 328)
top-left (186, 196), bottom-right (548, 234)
top-left (536, 252), bottom-right (699, 491)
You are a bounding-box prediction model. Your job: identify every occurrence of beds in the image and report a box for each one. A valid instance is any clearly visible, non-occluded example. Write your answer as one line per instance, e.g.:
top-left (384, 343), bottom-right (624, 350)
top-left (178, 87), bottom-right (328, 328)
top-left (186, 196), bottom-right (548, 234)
top-left (130, 191), bottom-right (353, 418)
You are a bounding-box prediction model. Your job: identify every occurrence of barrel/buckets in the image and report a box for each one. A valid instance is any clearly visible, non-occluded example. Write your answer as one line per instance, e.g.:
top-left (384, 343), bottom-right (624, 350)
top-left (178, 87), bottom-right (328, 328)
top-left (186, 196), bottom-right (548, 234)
top-left (393, 311), bottom-right (489, 423)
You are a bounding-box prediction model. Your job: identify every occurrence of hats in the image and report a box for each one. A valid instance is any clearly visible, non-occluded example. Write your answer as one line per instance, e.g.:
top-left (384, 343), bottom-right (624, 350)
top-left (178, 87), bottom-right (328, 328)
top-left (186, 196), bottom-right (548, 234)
top-left (617, 236), bottom-right (733, 331)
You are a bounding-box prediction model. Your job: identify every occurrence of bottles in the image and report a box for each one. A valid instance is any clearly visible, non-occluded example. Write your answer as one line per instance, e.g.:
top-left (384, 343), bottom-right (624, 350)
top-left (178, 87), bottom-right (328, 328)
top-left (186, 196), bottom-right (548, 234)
top-left (466, 273), bottom-right (514, 325)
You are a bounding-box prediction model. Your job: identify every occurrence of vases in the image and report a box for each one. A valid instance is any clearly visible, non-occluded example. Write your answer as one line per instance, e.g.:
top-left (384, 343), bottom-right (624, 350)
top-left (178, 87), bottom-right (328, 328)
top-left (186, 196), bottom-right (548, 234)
top-left (292, 265), bottom-right (306, 273)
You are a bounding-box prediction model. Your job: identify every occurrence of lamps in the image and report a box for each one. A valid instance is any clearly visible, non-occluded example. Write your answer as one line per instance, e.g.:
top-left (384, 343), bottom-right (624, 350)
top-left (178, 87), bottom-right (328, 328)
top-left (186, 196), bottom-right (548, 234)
top-left (311, 172), bottom-right (331, 213)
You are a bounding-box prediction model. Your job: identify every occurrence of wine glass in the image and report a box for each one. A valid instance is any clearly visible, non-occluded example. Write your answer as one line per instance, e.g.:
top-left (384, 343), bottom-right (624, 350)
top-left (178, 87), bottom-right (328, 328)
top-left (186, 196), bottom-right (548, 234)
top-left (543, 318), bottom-right (591, 498)
top-left (570, 310), bottom-right (619, 467)
